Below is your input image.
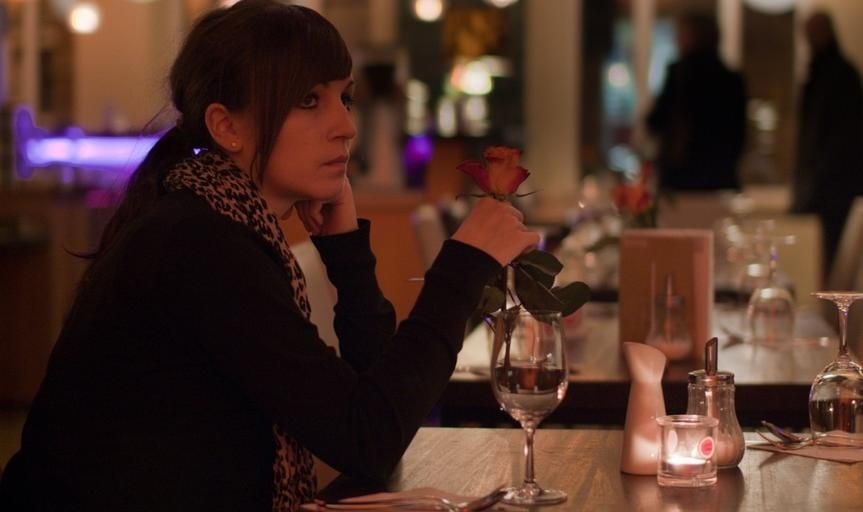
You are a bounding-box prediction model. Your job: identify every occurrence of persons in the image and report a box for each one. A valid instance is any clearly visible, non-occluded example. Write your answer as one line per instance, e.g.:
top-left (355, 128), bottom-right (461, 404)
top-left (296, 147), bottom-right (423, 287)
top-left (1, 3), bottom-right (542, 509)
top-left (790, 12), bottom-right (863, 286)
top-left (641, 8), bottom-right (750, 230)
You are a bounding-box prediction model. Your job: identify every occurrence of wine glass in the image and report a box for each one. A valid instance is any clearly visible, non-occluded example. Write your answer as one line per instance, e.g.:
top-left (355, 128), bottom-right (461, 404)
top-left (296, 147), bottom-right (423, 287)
top-left (747, 236), bottom-right (796, 341)
top-left (493, 309), bottom-right (569, 505)
top-left (807, 290), bottom-right (863, 450)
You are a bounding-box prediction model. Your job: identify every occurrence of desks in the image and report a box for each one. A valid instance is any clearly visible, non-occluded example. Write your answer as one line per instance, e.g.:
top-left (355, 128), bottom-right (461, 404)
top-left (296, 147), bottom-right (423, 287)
top-left (451, 304), bottom-right (862, 433)
top-left (387, 426), bottom-right (862, 510)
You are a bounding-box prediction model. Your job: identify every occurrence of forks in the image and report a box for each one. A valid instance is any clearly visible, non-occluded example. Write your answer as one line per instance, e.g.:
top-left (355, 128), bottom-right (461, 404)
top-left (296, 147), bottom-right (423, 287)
top-left (755, 428), bottom-right (862, 453)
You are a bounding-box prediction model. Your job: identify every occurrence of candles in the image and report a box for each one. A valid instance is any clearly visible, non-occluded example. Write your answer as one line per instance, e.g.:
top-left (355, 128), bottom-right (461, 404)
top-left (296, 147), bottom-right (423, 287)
top-left (654, 331), bottom-right (691, 362)
top-left (666, 451), bottom-right (708, 479)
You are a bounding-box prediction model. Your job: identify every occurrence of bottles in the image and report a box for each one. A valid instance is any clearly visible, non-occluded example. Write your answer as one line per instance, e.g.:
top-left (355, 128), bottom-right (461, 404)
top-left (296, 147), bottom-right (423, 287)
top-left (685, 336), bottom-right (744, 469)
top-left (621, 341), bottom-right (667, 475)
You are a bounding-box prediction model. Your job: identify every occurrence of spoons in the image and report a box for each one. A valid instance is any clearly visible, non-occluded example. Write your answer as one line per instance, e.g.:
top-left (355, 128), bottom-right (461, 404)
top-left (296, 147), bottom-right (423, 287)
top-left (762, 418), bottom-right (863, 443)
top-left (327, 490), bottom-right (507, 509)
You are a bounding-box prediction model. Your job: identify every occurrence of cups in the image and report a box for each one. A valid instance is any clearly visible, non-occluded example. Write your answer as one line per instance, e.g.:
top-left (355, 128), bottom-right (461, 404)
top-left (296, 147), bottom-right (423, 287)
top-left (618, 229), bottom-right (714, 372)
top-left (656, 415), bottom-right (718, 488)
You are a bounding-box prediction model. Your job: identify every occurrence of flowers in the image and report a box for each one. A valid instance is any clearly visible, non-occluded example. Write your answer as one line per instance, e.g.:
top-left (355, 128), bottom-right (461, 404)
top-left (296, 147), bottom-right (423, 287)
top-left (451, 144), bottom-right (594, 373)
